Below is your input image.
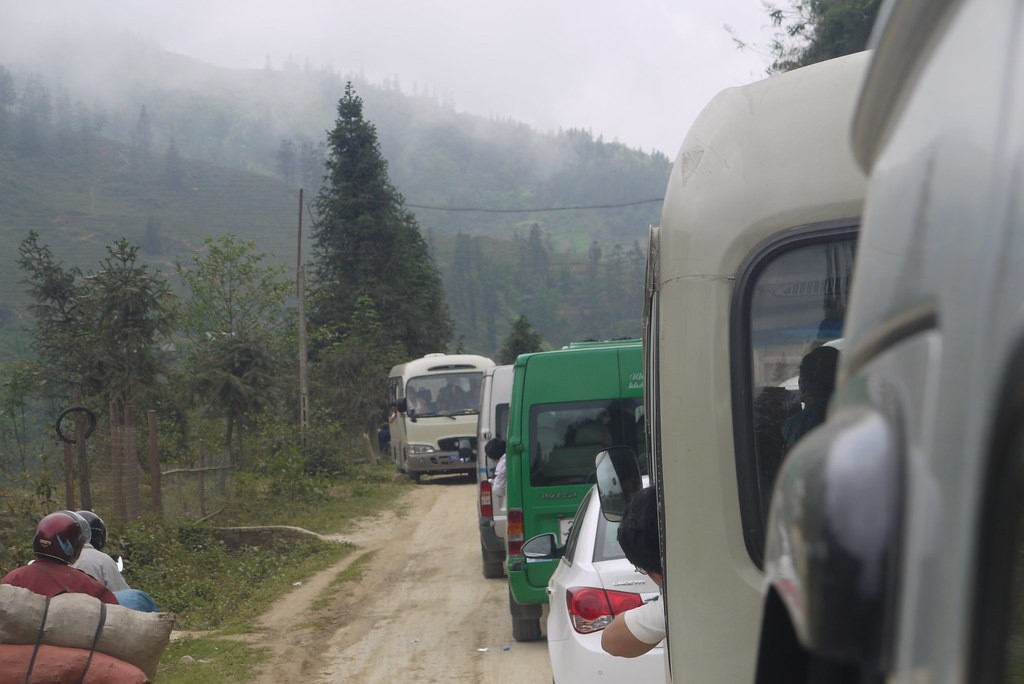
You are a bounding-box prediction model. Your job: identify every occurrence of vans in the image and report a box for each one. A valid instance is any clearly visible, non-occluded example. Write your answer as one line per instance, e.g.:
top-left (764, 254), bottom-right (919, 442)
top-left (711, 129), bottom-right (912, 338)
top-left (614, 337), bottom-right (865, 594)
top-left (608, 43), bottom-right (878, 684)
top-left (505, 336), bottom-right (650, 637)
top-left (472, 366), bottom-right (513, 574)
top-left (755, 1), bottom-right (1022, 684)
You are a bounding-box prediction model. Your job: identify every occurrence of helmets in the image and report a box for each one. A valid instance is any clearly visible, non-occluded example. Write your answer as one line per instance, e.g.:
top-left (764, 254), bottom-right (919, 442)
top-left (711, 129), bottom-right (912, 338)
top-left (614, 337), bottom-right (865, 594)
top-left (32, 509), bottom-right (91, 565)
top-left (76, 511), bottom-right (108, 551)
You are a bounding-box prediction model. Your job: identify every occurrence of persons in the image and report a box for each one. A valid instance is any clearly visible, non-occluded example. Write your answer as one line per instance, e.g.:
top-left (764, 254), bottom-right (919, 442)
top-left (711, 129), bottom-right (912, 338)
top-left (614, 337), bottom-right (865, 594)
top-left (0, 510), bottom-right (119, 606)
top-left (74, 510), bottom-right (131, 592)
top-left (485, 438), bottom-right (506, 495)
top-left (378, 422), bottom-right (391, 452)
top-left (386, 378), bottom-right (480, 419)
top-left (790, 344), bottom-right (840, 437)
top-left (598, 483), bottom-right (671, 661)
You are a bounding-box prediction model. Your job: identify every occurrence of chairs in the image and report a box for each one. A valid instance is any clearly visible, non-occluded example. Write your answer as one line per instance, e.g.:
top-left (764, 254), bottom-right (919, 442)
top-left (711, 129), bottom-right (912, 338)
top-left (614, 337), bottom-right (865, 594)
top-left (546, 444), bottom-right (604, 483)
top-left (571, 421), bottom-right (611, 446)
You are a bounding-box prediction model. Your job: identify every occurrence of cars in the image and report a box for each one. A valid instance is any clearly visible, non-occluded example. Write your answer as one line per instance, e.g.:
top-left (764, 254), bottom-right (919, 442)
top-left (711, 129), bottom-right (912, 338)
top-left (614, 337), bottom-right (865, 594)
top-left (518, 477), bottom-right (669, 683)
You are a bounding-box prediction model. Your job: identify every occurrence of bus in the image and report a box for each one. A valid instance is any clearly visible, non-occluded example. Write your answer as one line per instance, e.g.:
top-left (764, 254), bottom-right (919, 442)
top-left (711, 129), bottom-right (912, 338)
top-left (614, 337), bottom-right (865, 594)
top-left (382, 349), bottom-right (494, 481)
